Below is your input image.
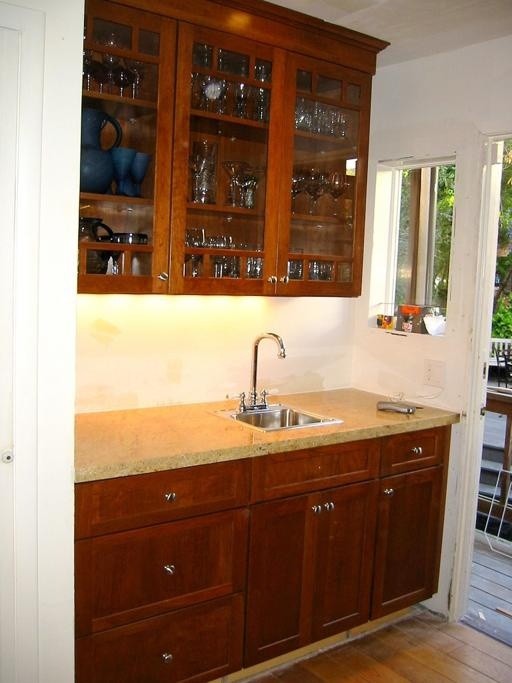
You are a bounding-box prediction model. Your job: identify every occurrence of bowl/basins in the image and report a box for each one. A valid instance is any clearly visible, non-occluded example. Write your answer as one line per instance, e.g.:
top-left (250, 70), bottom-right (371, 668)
top-left (112, 232), bottom-right (150, 244)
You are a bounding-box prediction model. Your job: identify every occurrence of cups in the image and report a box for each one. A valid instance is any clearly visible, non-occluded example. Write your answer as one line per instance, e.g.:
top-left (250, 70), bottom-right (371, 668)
top-left (377, 303), bottom-right (421, 336)
top-left (289, 247), bottom-right (351, 281)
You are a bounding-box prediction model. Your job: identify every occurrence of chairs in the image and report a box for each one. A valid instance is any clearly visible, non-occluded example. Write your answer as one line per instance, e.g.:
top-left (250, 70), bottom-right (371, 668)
top-left (494, 346), bottom-right (512, 389)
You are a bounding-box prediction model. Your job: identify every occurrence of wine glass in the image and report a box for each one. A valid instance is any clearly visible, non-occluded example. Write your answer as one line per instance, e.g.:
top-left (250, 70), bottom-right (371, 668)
top-left (84, 25), bottom-right (149, 101)
top-left (294, 97), bottom-right (352, 140)
top-left (192, 46), bottom-right (269, 123)
top-left (189, 140), bottom-right (350, 216)
top-left (186, 228), bottom-right (262, 280)
top-left (110, 146), bottom-right (153, 198)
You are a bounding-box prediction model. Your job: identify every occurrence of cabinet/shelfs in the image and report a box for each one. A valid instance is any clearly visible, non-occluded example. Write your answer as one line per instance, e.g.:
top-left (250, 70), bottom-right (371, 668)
top-left (73, 451), bottom-right (243, 683)
top-left (235, 429), bottom-right (382, 673)
top-left (167, 0), bottom-right (395, 303)
top-left (371, 417), bottom-right (452, 627)
top-left (71, 1), bottom-right (186, 301)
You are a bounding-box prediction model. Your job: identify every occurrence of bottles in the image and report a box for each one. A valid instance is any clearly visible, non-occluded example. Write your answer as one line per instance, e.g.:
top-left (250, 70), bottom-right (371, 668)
top-left (423, 306), bottom-right (442, 334)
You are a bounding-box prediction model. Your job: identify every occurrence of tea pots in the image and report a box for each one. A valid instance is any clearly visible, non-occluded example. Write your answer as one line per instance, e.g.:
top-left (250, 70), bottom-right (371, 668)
top-left (78, 214), bottom-right (114, 272)
top-left (80, 108), bottom-right (123, 193)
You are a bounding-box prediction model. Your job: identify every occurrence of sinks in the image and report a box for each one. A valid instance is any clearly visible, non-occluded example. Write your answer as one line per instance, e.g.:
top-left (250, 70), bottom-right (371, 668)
top-left (214, 402), bottom-right (343, 434)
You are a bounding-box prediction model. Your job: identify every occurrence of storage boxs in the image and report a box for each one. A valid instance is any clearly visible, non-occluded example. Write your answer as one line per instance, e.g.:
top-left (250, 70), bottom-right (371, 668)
top-left (396, 304), bottom-right (435, 334)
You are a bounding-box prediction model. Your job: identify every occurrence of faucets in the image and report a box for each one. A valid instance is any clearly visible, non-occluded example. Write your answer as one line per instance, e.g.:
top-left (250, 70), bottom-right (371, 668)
top-left (249, 332), bottom-right (287, 405)
